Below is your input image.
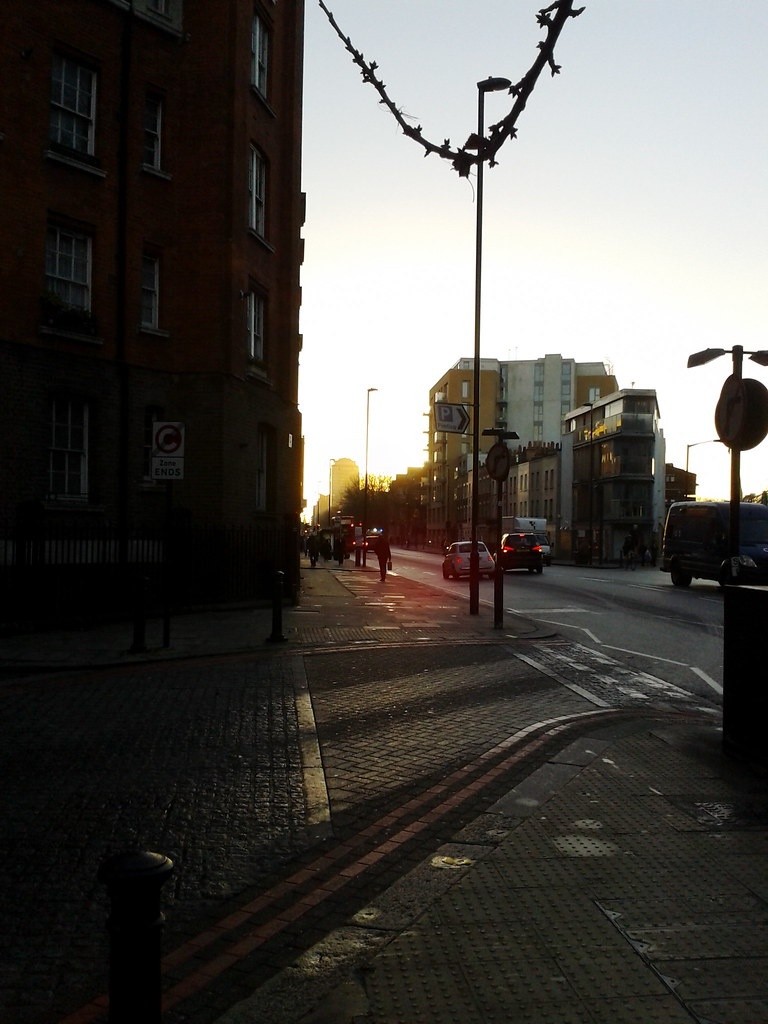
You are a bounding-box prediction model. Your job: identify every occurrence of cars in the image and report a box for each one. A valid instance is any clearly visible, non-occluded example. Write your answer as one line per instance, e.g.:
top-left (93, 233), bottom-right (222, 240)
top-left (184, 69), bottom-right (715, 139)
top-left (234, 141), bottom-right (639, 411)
top-left (442, 541), bottom-right (495, 579)
top-left (365, 529), bottom-right (381, 551)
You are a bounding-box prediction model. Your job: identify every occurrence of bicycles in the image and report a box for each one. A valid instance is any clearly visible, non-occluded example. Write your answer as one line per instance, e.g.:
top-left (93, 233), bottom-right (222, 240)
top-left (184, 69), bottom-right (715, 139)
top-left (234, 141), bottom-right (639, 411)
top-left (617, 547), bottom-right (638, 572)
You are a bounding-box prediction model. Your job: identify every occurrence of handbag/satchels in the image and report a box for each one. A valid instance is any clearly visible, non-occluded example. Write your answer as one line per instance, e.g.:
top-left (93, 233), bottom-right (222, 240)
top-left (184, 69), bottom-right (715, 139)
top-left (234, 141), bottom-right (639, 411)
top-left (388, 562), bottom-right (392, 570)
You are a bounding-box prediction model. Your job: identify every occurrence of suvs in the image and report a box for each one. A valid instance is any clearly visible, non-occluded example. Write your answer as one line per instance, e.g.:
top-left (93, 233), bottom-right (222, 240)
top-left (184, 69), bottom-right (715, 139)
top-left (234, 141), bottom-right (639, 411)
top-left (499, 532), bottom-right (545, 574)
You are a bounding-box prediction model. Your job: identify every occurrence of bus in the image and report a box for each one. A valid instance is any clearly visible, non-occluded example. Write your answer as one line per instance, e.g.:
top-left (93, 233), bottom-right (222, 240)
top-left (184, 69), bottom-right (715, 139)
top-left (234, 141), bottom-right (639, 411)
top-left (331, 511), bottom-right (364, 550)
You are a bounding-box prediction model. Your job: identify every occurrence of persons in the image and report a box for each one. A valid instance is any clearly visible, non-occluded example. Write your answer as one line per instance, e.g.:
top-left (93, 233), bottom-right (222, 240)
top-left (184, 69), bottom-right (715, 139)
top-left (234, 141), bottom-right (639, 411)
top-left (306, 534), bottom-right (344, 566)
top-left (638, 543), bottom-right (658, 567)
top-left (374, 535), bottom-right (391, 582)
top-left (623, 535), bottom-right (635, 564)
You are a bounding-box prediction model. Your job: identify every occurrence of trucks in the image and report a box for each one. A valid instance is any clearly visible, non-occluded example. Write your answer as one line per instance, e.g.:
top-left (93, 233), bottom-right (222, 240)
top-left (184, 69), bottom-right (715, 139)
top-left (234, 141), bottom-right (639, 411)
top-left (502, 516), bottom-right (555, 565)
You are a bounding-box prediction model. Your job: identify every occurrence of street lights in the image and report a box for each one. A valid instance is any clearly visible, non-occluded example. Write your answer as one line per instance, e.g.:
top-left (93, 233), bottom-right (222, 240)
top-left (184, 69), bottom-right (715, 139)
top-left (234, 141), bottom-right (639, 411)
top-left (469, 74), bottom-right (514, 615)
top-left (581, 402), bottom-right (595, 565)
top-left (328, 459), bottom-right (339, 525)
top-left (360, 387), bottom-right (379, 567)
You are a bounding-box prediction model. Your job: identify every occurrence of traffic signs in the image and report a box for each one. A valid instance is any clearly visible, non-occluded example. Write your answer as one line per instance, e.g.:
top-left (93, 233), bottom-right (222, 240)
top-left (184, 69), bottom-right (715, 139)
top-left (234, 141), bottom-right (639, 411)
top-left (434, 401), bottom-right (471, 435)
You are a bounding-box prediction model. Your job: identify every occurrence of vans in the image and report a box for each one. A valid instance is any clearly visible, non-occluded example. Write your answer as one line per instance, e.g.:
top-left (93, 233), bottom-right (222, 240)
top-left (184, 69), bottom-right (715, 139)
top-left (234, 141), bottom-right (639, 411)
top-left (662, 501), bottom-right (768, 586)
